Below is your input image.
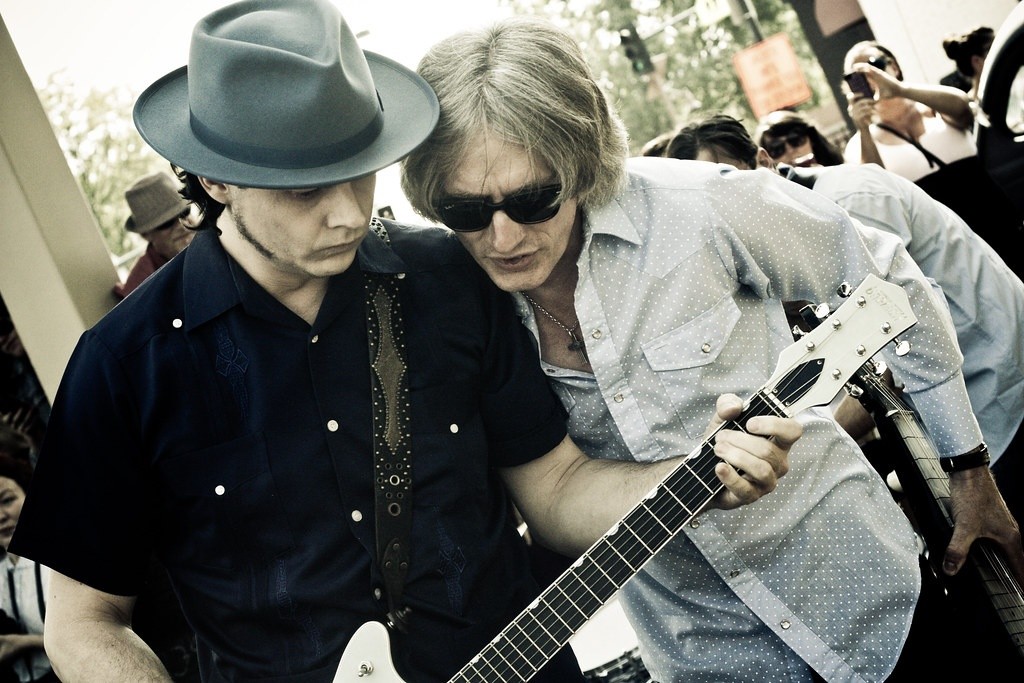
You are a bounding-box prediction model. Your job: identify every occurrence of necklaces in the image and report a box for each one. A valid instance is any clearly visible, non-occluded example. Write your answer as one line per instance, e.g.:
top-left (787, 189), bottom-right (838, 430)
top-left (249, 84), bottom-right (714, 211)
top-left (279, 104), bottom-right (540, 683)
top-left (519, 293), bottom-right (587, 367)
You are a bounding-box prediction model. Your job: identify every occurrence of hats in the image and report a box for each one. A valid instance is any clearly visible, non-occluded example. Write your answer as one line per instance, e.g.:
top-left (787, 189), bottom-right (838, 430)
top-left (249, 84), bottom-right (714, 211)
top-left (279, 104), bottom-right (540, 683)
top-left (133, 0), bottom-right (440, 190)
top-left (124, 171), bottom-right (193, 233)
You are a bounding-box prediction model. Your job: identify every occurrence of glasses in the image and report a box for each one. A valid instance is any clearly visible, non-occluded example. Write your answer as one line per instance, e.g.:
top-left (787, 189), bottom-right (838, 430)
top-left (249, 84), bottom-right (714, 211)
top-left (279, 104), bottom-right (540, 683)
top-left (157, 209), bottom-right (190, 231)
top-left (767, 132), bottom-right (808, 158)
top-left (869, 57), bottom-right (894, 71)
top-left (431, 185), bottom-right (563, 233)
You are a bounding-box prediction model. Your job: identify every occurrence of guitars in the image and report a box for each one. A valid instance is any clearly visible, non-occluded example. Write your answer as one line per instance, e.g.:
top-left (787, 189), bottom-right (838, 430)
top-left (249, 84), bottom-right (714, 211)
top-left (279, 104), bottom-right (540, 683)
top-left (326, 274), bottom-right (920, 683)
top-left (790, 300), bottom-right (1024, 660)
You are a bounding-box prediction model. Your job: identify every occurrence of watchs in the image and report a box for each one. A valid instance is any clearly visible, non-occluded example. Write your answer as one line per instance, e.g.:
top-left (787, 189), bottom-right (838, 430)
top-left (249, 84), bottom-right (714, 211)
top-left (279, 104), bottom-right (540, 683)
top-left (940, 442), bottom-right (990, 473)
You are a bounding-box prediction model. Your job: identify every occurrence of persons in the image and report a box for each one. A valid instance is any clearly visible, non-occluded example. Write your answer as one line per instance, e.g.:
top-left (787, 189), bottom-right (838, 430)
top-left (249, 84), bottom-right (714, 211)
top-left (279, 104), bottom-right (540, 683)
top-left (397, 21), bottom-right (1024, 683)
top-left (941, 30), bottom-right (1024, 136)
top-left (8, 1), bottom-right (802, 682)
top-left (843, 41), bottom-right (975, 182)
top-left (638, 111), bottom-right (1024, 536)
top-left (0, 430), bottom-right (61, 683)
top-left (121, 173), bottom-right (197, 298)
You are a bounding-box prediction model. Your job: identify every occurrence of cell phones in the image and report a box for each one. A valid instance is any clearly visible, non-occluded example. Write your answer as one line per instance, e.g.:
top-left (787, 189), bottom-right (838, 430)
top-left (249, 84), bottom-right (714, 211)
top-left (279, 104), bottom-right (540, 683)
top-left (845, 72), bottom-right (874, 103)
top-left (792, 153), bottom-right (816, 168)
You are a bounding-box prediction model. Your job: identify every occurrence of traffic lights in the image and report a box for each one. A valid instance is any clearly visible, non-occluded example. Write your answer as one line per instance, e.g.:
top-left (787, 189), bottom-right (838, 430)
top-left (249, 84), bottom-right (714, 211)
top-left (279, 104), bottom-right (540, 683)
top-left (619, 21), bottom-right (654, 75)
